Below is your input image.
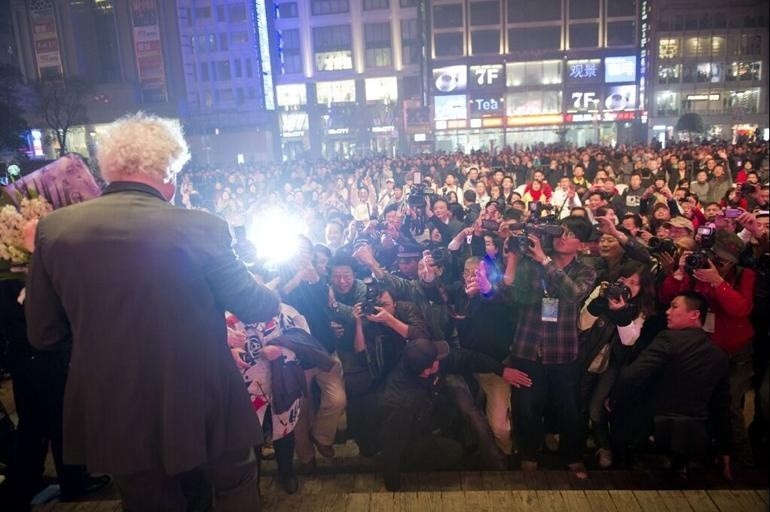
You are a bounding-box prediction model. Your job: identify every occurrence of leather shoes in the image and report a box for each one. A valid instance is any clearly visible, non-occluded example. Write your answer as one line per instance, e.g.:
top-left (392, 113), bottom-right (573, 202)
top-left (59, 475), bottom-right (110, 500)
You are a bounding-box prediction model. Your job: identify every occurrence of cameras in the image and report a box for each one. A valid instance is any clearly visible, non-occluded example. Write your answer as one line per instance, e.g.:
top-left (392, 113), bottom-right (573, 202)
top-left (360, 283), bottom-right (382, 315)
top-left (635, 231), bottom-right (644, 237)
top-left (654, 187), bottom-right (662, 192)
top-left (603, 280), bottom-right (631, 299)
top-left (431, 246), bottom-right (452, 266)
top-left (481, 219), bottom-right (500, 231)
top-left (374, 214), bottom-right (388, 230)
top-left (740, 181), bottom-right (757, 193)
top-left (683, 226), bottom-right (715, 275)
top-left (646, 235), bottom-right (677, 257)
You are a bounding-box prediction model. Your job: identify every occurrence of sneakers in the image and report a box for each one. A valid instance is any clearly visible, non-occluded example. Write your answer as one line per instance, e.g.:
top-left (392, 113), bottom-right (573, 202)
top-left (544, 433), bottom-right (559, 451)
top-left (595, 448), bottom-right (611, 466)
top-left (309, 428), bottom-right (335, 457)
top-left (567, 461), bottom-right (588, 480)
top-left (281, 471), bottom-right (298, 494)
top-left (299, 456), bottom-right (316, 475)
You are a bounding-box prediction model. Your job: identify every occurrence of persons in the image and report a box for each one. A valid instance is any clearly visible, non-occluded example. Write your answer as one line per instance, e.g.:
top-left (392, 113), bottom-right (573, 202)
top-left (0, 133), bottom-right (770, 511)
top-left (24, 106), bottom-right (281, 511)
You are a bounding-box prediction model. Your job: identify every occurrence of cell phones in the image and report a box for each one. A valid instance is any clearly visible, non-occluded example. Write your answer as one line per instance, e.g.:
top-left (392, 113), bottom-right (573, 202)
top-left (726, 209), bottom-right (741, 217)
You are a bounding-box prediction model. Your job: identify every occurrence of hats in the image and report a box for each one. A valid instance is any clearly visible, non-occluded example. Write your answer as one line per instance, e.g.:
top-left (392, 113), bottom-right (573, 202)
top-left (401, 338), bottom-right (450, 376)
top-left (393, 244), bottom-right (420, 263)
